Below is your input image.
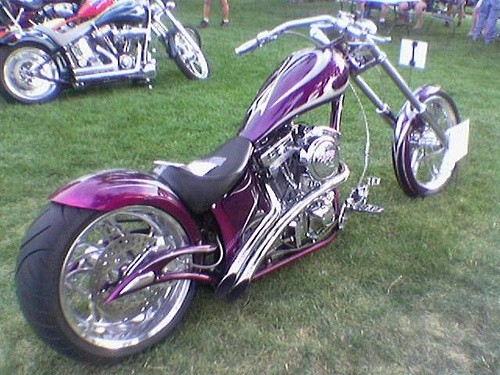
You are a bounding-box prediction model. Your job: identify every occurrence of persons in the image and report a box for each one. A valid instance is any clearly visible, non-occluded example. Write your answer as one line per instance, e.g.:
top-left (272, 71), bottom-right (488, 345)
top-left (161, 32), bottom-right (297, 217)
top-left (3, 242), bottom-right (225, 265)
top-left (356, 0), bottom-right (500, 44)
top-left (197, 0), bottom-right (231, 30)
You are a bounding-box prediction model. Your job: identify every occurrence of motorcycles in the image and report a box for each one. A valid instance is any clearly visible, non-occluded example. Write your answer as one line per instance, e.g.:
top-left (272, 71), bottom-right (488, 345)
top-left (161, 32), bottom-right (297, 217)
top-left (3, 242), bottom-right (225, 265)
top-left (2, 0), bottom-right (209, 107)
top-left (14, 9), bottom-right (470, 367)
